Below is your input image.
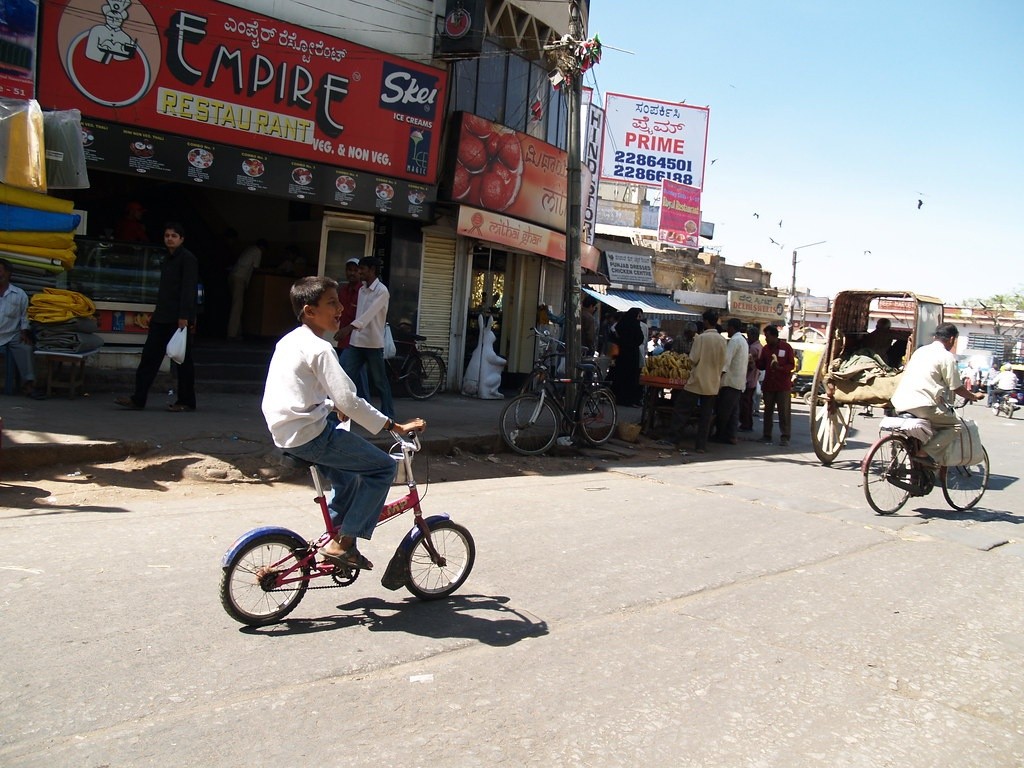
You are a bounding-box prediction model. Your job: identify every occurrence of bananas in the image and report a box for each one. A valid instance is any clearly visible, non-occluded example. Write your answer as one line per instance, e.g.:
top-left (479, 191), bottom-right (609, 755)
top-left (643, 350), bottom-right (692, 379)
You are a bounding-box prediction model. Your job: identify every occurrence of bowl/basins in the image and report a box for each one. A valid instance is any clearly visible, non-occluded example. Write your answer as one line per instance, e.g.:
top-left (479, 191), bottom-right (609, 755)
top-left (387, 441), bottom-right (414, 483)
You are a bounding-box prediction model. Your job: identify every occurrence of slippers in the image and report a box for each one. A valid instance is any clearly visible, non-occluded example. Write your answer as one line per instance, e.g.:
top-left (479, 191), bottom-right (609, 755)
top-left (318, 545), bottom-right (373, 571)
top-left (911, 455), bottom-right (938, 468)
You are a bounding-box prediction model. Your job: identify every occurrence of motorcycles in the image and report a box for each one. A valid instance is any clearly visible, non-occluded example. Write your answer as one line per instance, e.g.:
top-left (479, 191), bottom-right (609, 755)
top-left (787, 341), bottom-right (826, 406)
top-left (990, 383), bottom-right (1021, 418)
top-left (1000, 364), bottom-right (1024, 405)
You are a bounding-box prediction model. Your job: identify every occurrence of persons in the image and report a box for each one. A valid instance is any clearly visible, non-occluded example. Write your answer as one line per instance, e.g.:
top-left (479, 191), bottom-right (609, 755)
top-left (225, 238), bottom-right (271, 342)
top-left (739, 326), bottom-right (763, 432)
top-left (664, 309), bottom-right (727, 455)
top-left (670, 318), bottom-right (705, 355)
top-left (750, 323), bottom-right (794, 447)
top-left (209, 228), bottom-right (239, 335)
top-left (990, 363), bottom-right (1018, 408)
top-left (259, 273), bottom-right (426, 572)
top-left (333, 257), bottom-right (371, 403)
top-left (112, 221), bottom-right (199, 412)
top-left (113, 202), bottom-right (152, 242)
top-left (0, 257), bottom-right (49, 400)
top-left (960, 359), bottom-right (980, 403)
top-left (865, 318), bottom-right (911, 366)
top-left (713, 317), bottom-right (750, 445)
top-left (890, 323), bottom-right (986, 468)
top-left (326, 255), bottom-right (398, 422)
top-left (287, 243), bottom-right (314, 276)
top-left (598, 307), bottom-right (667, 407)
top-left (572, 294), bottom-right (598, 421)
top-left (981, 362), bottom-right (1000, 406)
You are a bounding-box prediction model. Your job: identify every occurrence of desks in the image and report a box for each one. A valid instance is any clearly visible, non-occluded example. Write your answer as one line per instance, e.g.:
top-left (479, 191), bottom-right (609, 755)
top-left (33, 349), bottom-right (100, 401)
top-left (639, 376), bottom-right (717, 437)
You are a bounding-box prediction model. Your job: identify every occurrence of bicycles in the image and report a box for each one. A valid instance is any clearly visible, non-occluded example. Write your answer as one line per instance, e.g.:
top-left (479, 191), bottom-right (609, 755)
top-left (383, 320), bottom-right (447, 401)
top-left (498, 326), bottom-right (619, 456)
top-left (218, 430), bottom-right (476, 628)
top-left (857, 397), bottom-right (990, 516)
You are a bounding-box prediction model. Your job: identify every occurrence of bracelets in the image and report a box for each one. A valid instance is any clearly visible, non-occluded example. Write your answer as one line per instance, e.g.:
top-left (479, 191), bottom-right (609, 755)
top-left (385, 418), bottom-right (394, 431)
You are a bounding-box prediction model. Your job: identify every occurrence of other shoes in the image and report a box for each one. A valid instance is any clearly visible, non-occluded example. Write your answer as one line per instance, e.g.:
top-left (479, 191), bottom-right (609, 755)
top-left (696, 448), bottom-right (704, 454)
top-left (782, 440), bottom-right (790, 446)
top-left (168, 402), bottom-right (195, 411)
top-left (709, 434), bottom-right (737, 445)
top-left (738, 427), bottom-right (752, 432)
top-left (114, 395), bottom-right (144, 410)
top-left (22, 387), bottom-right (41, 400)
top-left (758, 436), bottom-right (772, 443)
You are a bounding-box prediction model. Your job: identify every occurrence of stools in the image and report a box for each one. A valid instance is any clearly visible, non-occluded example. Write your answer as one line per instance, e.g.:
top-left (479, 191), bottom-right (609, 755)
top-left (359, 361), bottom-right (372, 404)
top-left (0, 346), bottom-right (21, 396)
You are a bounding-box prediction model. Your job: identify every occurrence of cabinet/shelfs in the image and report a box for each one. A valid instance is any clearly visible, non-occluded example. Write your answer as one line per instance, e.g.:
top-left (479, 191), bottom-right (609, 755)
top-left (67, 237), bottom-right (204, 337)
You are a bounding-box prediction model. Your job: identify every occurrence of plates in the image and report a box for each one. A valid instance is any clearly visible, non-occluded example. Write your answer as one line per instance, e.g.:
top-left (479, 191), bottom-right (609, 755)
top-left (131, 141), bottom-right (155, 158)
top-left (684, 220), bottom-right (697, 234)
top-left (188, 148), bottom-right (213, 168)
top-left (375, 183), bottom-right (394, 200)
top-left (336, 176), bottom-right (357, 193)
top-left (82, 138), bottom-right (94, 146)
top-left (242, 159), bottom-right (264, 177)
top-left (407, 189), bottom-right (424, 205)
top-left (292, 168), bottom-right (312, 185)
top-left (667, 239), bottom-right (684, 243)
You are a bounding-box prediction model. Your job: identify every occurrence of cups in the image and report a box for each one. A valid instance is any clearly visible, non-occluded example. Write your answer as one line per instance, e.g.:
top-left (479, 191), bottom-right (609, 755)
top-left (687, 241), bottom-right (692, 246)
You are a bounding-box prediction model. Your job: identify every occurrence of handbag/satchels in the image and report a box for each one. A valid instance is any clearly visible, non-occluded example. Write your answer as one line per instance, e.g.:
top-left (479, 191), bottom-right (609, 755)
top-left (383, 323), bottom-right (396, 359)
top-left (935, 413), bottom-right (984, 466)
top-left (167, 326), bottom-right (188, 365)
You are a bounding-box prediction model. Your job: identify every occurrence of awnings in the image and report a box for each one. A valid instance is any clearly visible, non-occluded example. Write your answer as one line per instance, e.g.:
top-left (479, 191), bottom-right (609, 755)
top-left (581, 283), bottom-right (702, 319)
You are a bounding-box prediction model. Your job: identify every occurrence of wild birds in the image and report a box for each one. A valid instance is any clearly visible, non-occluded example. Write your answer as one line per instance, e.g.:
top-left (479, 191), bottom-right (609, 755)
top-left (711, 159), bottom-right (717, 165)
top-left (779, 220), bottom-right (782, 228)
top-left (864, 251), bottom-right (871, 254)
top-left (768, 237), bottom-right (779, 245)
top-left (753, 213), bottom-right (759, 219)
top-left (918, 199), bottom-right (923, 209)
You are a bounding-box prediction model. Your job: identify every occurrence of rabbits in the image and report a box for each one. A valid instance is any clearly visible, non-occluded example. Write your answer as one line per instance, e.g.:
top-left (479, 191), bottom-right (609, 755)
top-left (462, 312), bottom-right (507, 400)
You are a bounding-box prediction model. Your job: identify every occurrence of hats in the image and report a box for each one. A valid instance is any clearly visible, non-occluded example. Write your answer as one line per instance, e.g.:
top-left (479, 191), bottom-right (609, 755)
top-left (1005, 364), bottom-right (1011, 371)
top-left (346, 258), bottom-right (360, 265)
